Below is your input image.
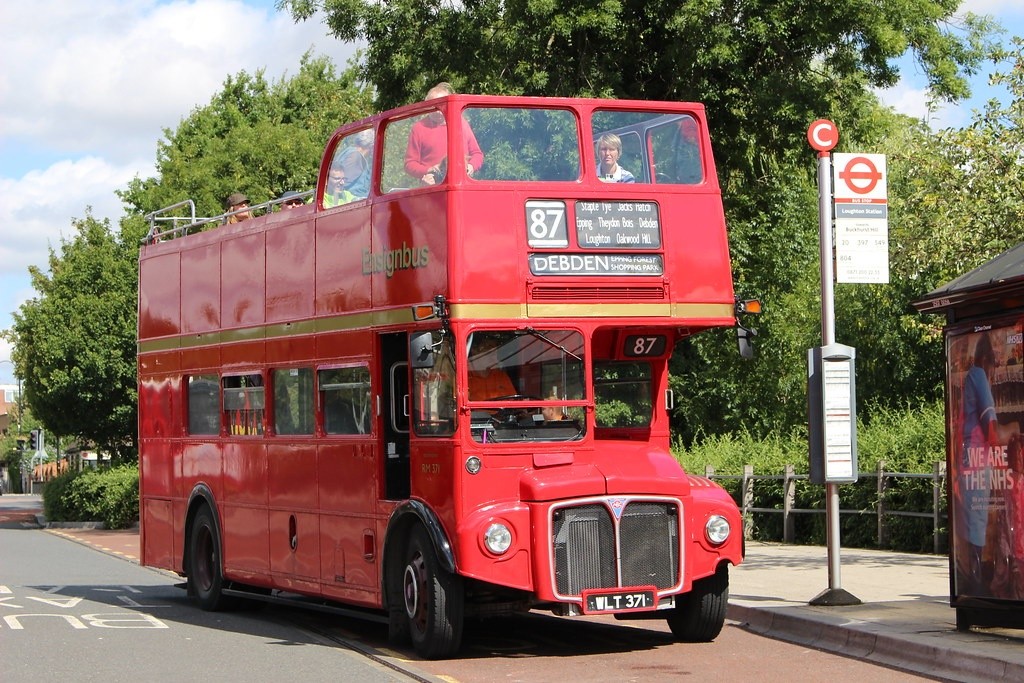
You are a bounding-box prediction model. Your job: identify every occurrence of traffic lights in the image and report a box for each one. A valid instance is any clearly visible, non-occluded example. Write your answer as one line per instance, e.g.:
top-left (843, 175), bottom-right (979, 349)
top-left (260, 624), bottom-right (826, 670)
top-left (30, 430), bottom-right (39, 451)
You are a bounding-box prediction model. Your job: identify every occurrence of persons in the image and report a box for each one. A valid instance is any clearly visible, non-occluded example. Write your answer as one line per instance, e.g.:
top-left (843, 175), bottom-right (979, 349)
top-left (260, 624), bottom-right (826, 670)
top-left (225, 193), bottom-right (255, 224)
top-left (954, 331), bottom-right (1023, 599)
top-left (322, 126), bottom-right (376, 209)
top-left (404, 82), bottom-right (485, 187)
top-left (323, 375), bottom-right (371, 435)
top-left (281, 191), bottom-right (305, 211)
top-left (468, 337), bottom-right (528, 416)
top-left (596, 133), bottom-right (636, 184)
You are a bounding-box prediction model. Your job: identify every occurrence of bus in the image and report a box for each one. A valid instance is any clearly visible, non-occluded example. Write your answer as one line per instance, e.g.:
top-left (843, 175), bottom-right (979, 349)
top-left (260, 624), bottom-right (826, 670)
top-left (135, 92), bottom-right (760, 661)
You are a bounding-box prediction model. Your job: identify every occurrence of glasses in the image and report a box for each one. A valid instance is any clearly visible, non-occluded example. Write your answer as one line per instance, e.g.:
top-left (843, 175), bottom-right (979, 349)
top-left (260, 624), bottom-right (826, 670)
top-left (285, 200), bottom-right (302, 205)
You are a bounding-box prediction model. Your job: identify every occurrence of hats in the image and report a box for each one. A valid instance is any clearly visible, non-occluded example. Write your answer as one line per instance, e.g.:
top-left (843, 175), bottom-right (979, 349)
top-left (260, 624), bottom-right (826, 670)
top-left (282, 191), bottom-right (304, 202)
top-left (225, 194), bottom-right (251, 208)
